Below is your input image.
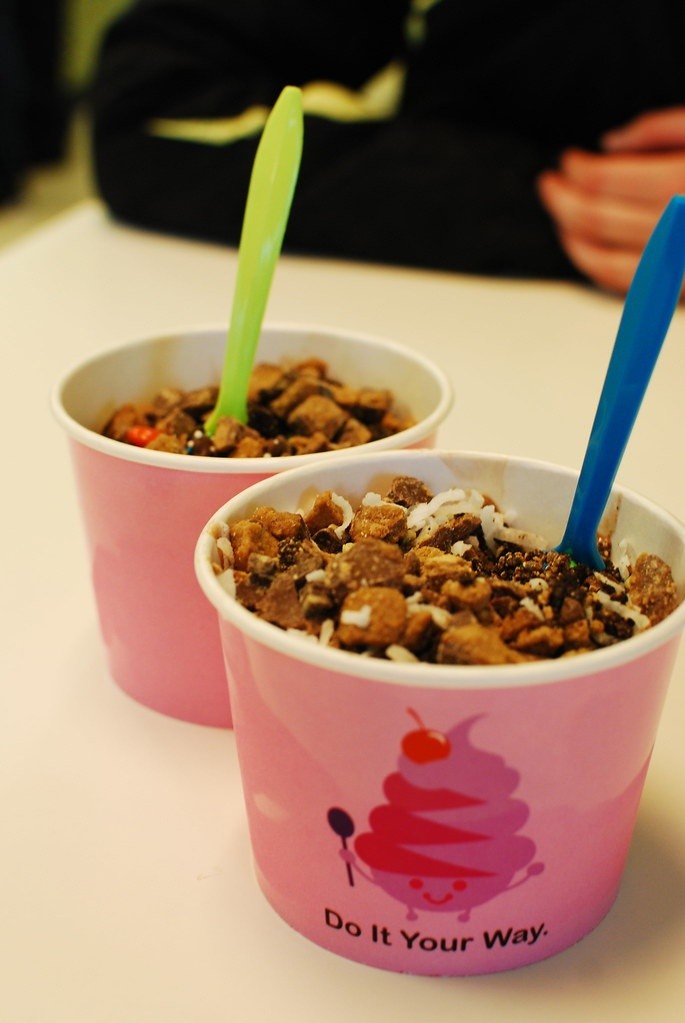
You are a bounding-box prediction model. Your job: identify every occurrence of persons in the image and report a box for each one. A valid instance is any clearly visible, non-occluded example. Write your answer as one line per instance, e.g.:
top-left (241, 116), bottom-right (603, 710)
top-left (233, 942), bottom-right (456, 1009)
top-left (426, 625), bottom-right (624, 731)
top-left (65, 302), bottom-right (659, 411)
top-left (1, 1), bottom-right (76, 213)
top-left (86, 1), bottom-right (685, 307)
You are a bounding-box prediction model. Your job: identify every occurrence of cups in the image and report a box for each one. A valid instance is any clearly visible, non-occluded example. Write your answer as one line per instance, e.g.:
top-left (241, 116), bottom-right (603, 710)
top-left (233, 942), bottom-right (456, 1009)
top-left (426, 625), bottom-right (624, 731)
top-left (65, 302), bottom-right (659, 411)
top-left (55, 322), bottom-right (453, 729)
top-left (204, 447), bottom-right (685, 982)
